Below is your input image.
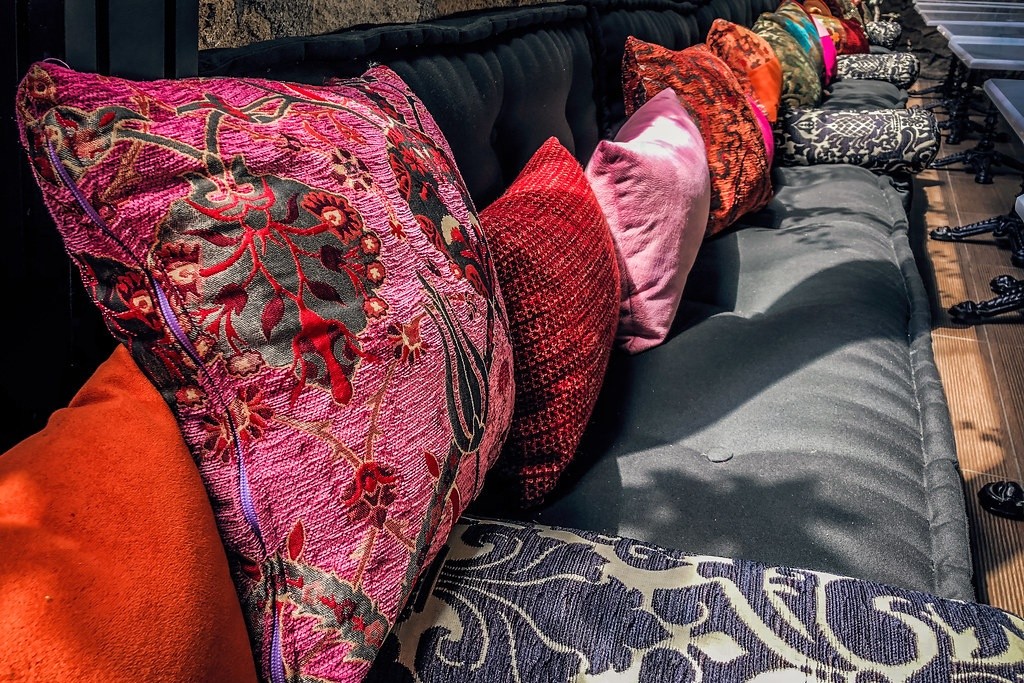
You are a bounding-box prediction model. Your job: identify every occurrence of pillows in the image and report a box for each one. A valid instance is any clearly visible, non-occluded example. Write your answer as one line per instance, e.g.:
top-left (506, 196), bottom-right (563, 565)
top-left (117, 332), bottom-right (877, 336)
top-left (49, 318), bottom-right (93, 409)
top-left (583, 87), bottom-right (712, 355)
top-left (745, 94), bottom-right (774, 165)
top-left (750, 14), bottom-right (821, 109)
top-left (705, 18), bottom-right (783, 125)
top-left (803, 0), bottom-right (832, 16)
top-left (840, 16), bottom-right (871, 54)
top-left (759, 0), bottom-right (825, 75)
top-left (811, 13), bottom-right (846, 54)
top-left (0, 340), bottom-right (258, 683)
top-left (16, 60), bottom-right (518, 683)
top-left (621, 35), bottom-right (774, 239)
top-left (812, 17), bottom-right (837, 86)
top-left (478, 135), bottom-right (622, 510)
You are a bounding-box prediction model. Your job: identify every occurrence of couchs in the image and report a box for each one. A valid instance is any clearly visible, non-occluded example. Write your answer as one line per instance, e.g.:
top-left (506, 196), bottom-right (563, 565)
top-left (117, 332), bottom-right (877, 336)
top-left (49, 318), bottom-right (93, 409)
top-left (0, 0), bottom-right (975, 683)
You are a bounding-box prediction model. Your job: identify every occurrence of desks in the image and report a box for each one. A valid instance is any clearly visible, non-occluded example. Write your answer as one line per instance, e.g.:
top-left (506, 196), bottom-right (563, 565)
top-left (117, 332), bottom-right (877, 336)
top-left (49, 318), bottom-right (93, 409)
top-left (926, 40), bottom-right (1024, 184)
top-left (901, 0), bottom-right (1024, 32)
top-left (922, 23), bottom-right (1024, 146)
top-left (930, 78), bottom-right (1024, 268)
top-left (898, 12), bottom-right (1024, 101)
top-left (910, 4), bottom-right (1024, 71)
